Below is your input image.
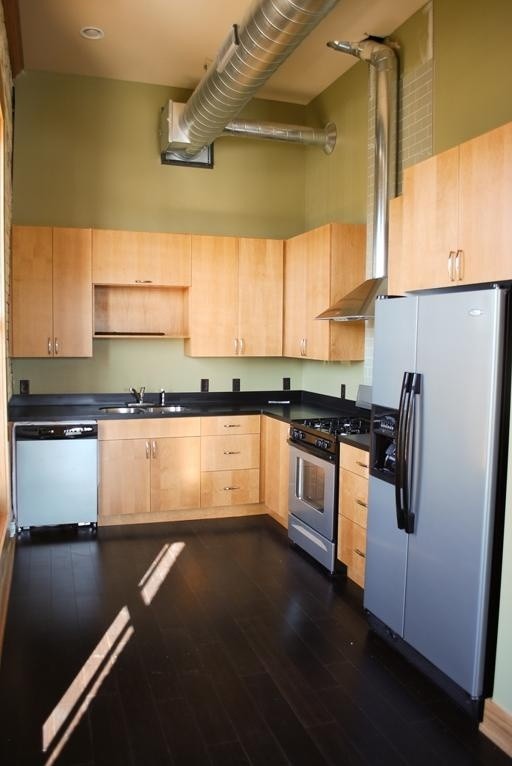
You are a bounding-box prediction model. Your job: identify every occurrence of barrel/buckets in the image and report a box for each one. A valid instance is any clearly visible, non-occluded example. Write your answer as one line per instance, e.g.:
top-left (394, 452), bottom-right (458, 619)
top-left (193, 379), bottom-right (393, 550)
top-left (130, 387), bottom-right (143, 403)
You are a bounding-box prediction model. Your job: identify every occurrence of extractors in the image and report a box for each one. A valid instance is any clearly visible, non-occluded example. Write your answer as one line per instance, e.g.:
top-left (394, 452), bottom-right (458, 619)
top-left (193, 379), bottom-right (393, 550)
top-left (314, 277), bottom-right (388, 321)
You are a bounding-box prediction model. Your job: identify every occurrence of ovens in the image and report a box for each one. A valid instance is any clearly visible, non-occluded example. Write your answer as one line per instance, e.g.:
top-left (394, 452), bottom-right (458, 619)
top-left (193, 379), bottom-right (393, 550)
top-left (285, 428), bottom-right (340, 577)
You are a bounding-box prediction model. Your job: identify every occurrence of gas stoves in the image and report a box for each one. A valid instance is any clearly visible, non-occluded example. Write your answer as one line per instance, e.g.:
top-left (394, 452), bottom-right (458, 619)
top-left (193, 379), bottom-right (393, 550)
top-left (289, 413), bottom-right (370, 443)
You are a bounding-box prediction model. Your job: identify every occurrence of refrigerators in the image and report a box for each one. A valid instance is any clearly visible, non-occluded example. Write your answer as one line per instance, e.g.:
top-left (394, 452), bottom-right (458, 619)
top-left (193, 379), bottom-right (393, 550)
top-left (360, 286), bottom-right (508, 716)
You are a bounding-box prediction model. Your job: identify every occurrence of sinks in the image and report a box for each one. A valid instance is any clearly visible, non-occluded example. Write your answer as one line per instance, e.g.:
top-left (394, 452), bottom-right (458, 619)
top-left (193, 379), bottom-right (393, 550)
top-left (99, 406), bottom-right (147, 414)
top-left (147, 405), bottom-right (198, 414)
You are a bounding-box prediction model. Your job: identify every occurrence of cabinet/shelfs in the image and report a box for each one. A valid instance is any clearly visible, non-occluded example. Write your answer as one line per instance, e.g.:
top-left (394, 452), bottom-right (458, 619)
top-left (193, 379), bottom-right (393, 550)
top-left (10, 224), bottom-right (91, 358)
top-left (401, 119), bottom-right (512, 294)
top-left (284, 220), bottom-right (366, 360)
top-left (185, 235), bottom-right (284, 358)
top-left (337, 440), bottom-right (370, 588)
top-left (201, 413), bottom-right (259, 520)
top-left (262, 412), bottom-right (288, 527)
top-left (388, 196), bottom-right (407, 296)
top-left (94, 229), bottom-right (189, 340)
top-left (99, 418), bottom-right (199, 523)
